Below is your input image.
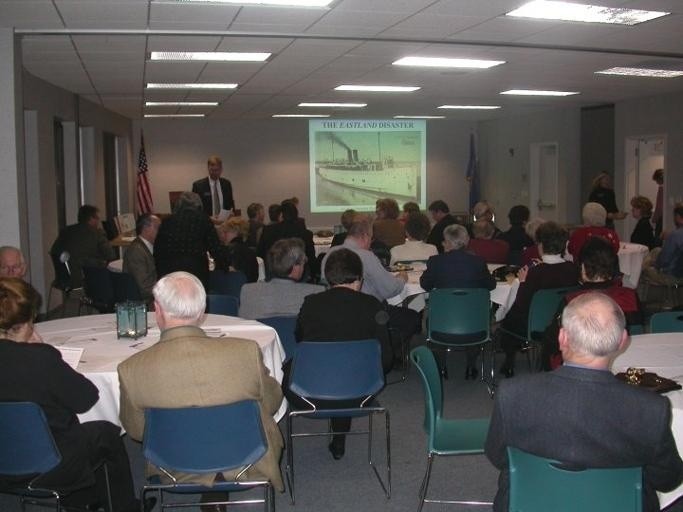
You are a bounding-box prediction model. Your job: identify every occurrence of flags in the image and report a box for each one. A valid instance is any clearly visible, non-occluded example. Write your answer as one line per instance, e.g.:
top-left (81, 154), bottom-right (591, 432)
top-left (137, 133), bottom-right (154, 216)
top-left (464, 129), bottom-right (482, 214)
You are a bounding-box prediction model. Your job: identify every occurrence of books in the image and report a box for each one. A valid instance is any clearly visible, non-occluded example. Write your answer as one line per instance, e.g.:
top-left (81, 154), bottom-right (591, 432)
top-left (52, 346), bottom-right (84, 368)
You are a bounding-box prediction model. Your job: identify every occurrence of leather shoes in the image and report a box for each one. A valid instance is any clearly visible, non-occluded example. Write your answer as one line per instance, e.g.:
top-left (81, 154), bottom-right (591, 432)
top-left (465, 367), bottom-right (478, 380)
top-left (440, 367), bottom-right (448, 380)
top-left (133, 496), bottom-right (157, 512)
top-left (201, 504), bottom-right (226, 512)
top-left (329, 440), bottom-right (345, 459)
top-left (500, 364), bottom-right (514, 378)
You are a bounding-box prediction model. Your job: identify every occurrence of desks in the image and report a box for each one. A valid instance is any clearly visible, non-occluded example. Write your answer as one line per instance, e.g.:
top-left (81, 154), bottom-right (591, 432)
top-left (107, 254), bottom-right (266, 273)
top-left (561, 241), bottom-right (649, 263)
top-left (375, 264), bottom-right (531, 309)
top-left (307, 235), bottom-right (337, 256)
top-left (22, 314), bottom-right (276, 463)
top-left (613, 333), bottom-right (683, 495)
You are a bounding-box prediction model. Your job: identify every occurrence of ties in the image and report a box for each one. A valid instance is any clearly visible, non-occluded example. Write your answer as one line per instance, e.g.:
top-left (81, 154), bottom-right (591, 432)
top-left (213, 179), bottom-right (220, 215)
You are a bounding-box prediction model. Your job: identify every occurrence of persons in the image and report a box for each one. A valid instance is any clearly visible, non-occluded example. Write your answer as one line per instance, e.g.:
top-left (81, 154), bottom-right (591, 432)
top-left (281, 248), bottom-right (395, 460)
top-left (544, 236), bottom-right (642, 379)
top-left (246, 203), bottom-right (265, 241)
top-left (49, 207), bottom-right (105, 291)
top-left (320, 224), bottom-right (408, 302)
top-left (0, 277), bottom-right (157, 511)
top-left (263, 205), bottom-right (283, 235)
top-left (153, 191), bottom-right (236, 293)
top-left (567, 202), bottom-right (619, 264)
top-left (0, 246), bottom-right (27, 279)
top-left (238, 239), bottom-right (326, 319)
top-left (484, 291), bottom-right (683, 512)
top-left (400, 201), bottom-right (420, 222)
top-left (587, 173), bottom-right (628, 230)
top-left (630, 196), bottom-right (653, 245)
top-left (122, 214), bottom-right (162, 312)
top-left (118, 271), bottom-right (286, 512)
top-left (221, 216), bottom-right (258, 282)
top-left (385, 212), bottom-right (440, 306)
top-left (331, 210), bottom-right (374, 251)
top-left (500, 220), bottom-right (581, 379)
top-left (427, 200), bottom-right (458, 254)
top-left (652, 169), bottom-right (668, 238)
top-left (372, 199), bottom-right (406, 248)
top-left (497, 205), bottom-right (536, 266)
top-left (62, 205), bottom-right (121, 298)
top-left (420, 223), bottom-right (497, 381)
top-left (193, 157), bottom-right (235, 215)
top-left (639, 204), bottom-right (683, 299)
top-left (259, 203), bottom-right (315, 257)
top-left (465, 201), bottom-right (503, 241)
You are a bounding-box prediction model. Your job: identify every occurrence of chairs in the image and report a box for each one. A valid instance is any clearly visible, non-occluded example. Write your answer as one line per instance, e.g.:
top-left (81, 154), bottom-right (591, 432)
top-left (425, 288), bottom-right (495, 400)
top-left (204, 295), bottom-right (240, 317)
top-left (140, 401), bottom-right (274, 511)
top-left (281, 340), bottom-right (392, 499)
top-left (651, 311), bottom-right (683, 332)
top-left (254, 318), bottom-right (298, 366)
top-left (99, 271), bottom-right (140, 307)
top-left (410, 347), bottom-right (494, 512)
top-left (505, 446), bottom-right (641, 512)
top-left (101, 221), bottom-right (118, 240)
top-left (47, 253), bottom-right (84, 318)
top-left (1, 401), bottom-right (113, 511)
top-left (497, 287), bottom-right (586, 376)
top-left (78, 267), bottom-right (111, 313)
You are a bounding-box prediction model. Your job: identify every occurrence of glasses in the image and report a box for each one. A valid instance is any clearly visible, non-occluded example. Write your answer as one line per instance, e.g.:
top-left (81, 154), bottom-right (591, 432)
top-left (362, 231), bottom-right (374, 243)
